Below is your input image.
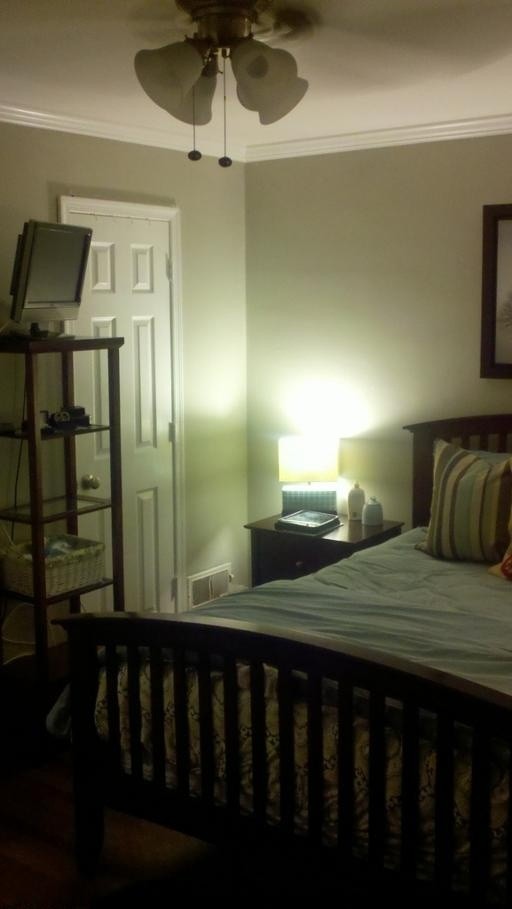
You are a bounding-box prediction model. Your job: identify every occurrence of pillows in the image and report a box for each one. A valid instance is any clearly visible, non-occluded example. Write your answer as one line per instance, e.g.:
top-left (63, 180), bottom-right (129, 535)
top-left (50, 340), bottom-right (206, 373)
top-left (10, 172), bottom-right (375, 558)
top-left (414, 437), bottom-right (512, 564)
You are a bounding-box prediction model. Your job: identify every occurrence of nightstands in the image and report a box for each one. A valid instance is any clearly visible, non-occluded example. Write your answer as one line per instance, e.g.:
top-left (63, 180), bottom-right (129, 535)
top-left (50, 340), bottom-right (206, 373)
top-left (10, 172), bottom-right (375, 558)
top-left (245, 511), bottom-right (404, 587)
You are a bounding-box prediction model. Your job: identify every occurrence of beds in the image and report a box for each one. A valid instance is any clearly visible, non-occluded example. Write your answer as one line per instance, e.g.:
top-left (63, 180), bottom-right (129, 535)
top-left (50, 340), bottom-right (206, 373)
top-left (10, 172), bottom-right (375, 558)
top-left (49, 413), bottom-right (512, 909)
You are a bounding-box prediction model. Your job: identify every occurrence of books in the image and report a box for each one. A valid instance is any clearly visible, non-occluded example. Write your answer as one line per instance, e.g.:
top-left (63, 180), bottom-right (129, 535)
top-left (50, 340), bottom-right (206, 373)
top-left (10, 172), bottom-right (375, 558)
top-left (274, 510), bottom-right (345, 537)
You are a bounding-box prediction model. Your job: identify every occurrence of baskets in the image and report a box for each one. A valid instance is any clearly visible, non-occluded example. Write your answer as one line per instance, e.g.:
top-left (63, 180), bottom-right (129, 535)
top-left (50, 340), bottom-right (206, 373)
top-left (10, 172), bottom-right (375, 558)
top-left (1, 531), bottom-right (108, 598)
top-left (1, 601), bottom-right (36, 663)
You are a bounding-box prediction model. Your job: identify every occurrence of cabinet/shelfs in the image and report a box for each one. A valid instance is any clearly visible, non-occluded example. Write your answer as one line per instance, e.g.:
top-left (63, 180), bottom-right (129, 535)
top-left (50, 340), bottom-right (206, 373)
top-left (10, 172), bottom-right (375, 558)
top-left (0, 338), bottom-right (126, 756)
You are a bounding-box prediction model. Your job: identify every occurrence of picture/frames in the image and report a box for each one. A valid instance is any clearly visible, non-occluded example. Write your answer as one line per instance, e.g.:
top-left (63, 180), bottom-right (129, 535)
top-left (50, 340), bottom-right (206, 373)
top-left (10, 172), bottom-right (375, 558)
top-left (480, 203), bottom-right (512, 379)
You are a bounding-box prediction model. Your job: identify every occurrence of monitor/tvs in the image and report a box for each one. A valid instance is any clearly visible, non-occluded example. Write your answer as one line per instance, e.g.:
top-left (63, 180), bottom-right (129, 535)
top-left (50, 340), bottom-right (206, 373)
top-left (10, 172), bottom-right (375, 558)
top-left (9, 218), bottom-right (94, 340)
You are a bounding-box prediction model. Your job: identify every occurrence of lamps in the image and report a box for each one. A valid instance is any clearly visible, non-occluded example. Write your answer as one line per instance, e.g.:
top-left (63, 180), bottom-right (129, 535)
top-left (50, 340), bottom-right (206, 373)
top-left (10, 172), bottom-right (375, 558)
top-left (133, 0), bottom-right (310, 167)
top-left (276, 434), bottom-right (341, 514)
